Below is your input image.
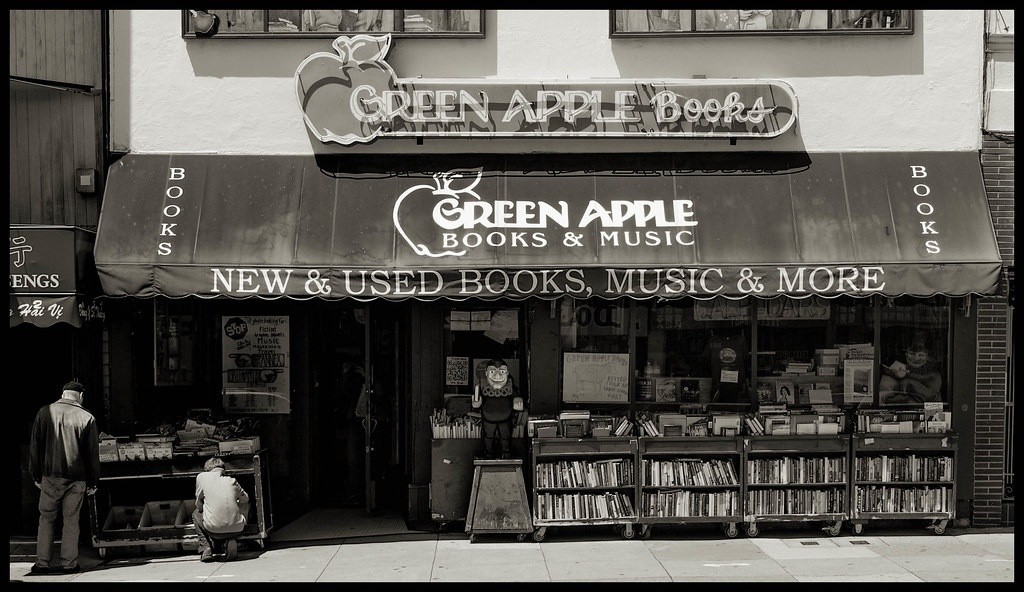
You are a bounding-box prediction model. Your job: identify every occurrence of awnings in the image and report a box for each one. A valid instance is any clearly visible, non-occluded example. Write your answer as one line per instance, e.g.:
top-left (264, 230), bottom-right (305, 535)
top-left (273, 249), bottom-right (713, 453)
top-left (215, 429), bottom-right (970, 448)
top-left (10, 152), bottom-right (1004, 330)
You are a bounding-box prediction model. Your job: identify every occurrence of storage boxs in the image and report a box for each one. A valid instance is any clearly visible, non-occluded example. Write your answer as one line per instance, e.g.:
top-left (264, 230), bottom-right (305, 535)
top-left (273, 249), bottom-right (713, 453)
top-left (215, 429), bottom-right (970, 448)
top-left (102, 499), bottom-right (200, 555)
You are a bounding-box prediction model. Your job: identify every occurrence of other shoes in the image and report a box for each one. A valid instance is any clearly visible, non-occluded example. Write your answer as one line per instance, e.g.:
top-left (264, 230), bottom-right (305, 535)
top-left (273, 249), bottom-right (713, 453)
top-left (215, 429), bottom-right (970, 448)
top-left (62, 563), bottom-right (80, 574)
top-left (224, 537), bottom-right (239, 562)
top-left (200, 548), bottom-right (214, 562)
top-left (31, 564), bottom-right (50, 574)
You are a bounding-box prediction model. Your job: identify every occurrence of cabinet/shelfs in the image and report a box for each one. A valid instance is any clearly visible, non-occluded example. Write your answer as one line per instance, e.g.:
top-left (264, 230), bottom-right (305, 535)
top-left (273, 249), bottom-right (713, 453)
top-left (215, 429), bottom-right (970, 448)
top-left (86, 448), bottom-right (274, 559)
top-left (464, 432), bottom-right (961, 543)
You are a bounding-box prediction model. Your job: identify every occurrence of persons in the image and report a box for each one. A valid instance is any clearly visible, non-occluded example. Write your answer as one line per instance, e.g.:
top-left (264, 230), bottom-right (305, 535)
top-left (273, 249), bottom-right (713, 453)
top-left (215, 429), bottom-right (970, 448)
top-left (28, 381), bottom-right (101, 575)
top-left (778, 386), bottom-right (792, 403)
top-left (191, 457), bottom-right (251, 562)
top-left (471, 359), bottom-right (526, 460)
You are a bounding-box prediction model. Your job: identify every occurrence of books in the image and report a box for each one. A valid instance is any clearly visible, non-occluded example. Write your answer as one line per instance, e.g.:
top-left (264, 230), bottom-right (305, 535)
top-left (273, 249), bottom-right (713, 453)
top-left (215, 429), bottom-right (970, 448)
top-left (430, 343), bottom-right (954, 514)
top-left (94, 419), bottom-right (264, 462)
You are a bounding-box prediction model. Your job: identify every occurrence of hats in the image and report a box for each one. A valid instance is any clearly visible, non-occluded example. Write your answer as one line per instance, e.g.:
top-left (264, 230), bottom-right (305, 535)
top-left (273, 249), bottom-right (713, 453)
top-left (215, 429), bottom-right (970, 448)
top-left (62, 380), bottom-right (86, 393)
